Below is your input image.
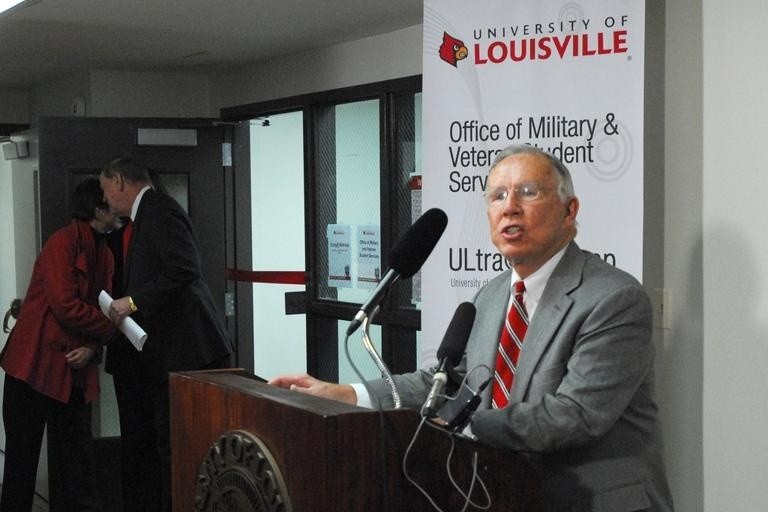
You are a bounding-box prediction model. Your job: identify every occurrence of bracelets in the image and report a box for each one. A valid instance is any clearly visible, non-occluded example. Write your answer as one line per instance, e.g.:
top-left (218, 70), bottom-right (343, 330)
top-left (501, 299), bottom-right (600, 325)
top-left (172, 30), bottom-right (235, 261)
top-left (129, 297), bottom-right (138, 313)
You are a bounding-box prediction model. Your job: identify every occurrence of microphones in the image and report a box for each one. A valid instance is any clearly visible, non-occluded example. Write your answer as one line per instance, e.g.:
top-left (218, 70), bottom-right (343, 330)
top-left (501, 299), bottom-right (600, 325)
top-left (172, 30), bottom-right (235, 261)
top-left (422, 301), bottom-right (477, 418)
top-left (347, 207), bottom-right (449, 335)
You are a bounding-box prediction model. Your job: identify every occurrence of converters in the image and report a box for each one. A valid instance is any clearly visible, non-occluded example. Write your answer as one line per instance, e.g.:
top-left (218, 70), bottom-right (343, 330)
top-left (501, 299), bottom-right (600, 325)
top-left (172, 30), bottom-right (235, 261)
top-left (432, 382), bottom-right (482, 432)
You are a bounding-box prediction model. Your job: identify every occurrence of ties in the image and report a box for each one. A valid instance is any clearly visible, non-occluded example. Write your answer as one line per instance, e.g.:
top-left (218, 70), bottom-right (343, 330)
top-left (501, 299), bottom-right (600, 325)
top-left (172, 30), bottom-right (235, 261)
top-left (122, 219), bottom-right (134, 270)
top-left (491, 281), bottom-right (529, 410)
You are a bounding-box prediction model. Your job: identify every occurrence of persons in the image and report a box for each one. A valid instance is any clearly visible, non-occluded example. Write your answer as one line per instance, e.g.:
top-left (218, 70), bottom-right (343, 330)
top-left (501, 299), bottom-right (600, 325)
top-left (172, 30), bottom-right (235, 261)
top-left (0, 174), bottom-right (122, 512)
top-left (98, 154), bottom-right (235, 512)
top-left (267, 142), bottom-right (677, 512)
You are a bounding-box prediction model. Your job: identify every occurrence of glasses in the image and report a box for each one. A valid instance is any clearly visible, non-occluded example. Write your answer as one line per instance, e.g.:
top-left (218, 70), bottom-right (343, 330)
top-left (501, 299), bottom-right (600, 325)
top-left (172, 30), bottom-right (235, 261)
top-left (482, 184), bottom-right (565, 202)
